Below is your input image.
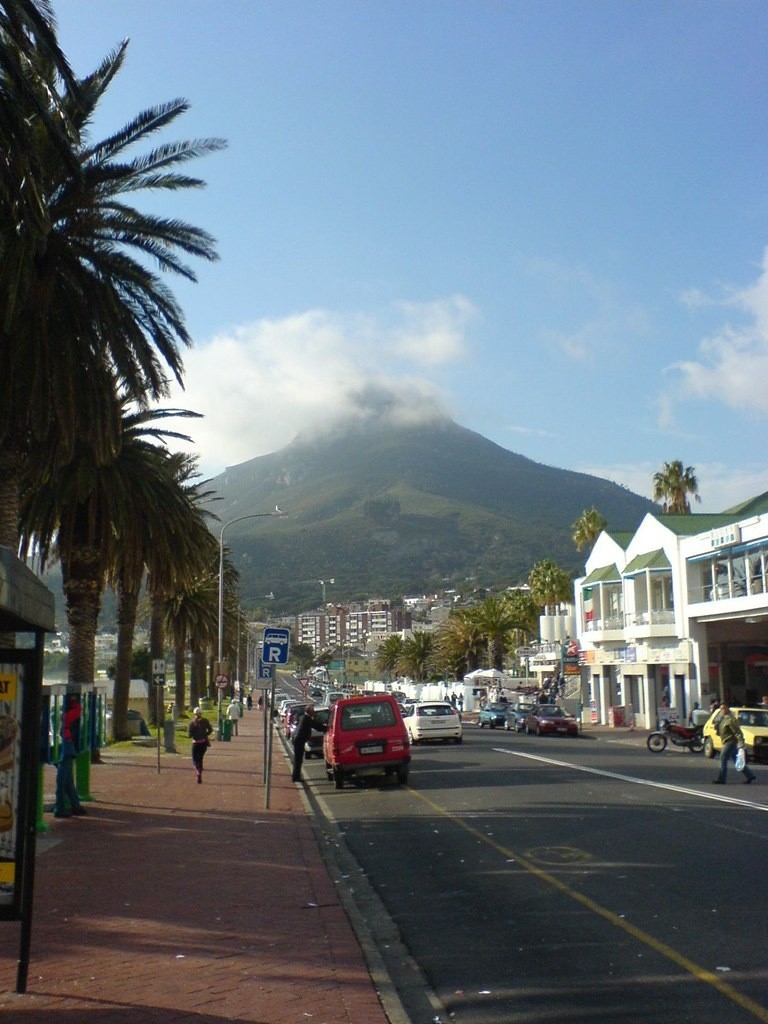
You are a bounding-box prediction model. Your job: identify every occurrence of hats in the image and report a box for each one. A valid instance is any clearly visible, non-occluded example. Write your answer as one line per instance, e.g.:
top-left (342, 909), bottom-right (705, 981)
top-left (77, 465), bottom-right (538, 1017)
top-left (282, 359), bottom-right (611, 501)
top-left (194, 707), bottom-right (202, 714)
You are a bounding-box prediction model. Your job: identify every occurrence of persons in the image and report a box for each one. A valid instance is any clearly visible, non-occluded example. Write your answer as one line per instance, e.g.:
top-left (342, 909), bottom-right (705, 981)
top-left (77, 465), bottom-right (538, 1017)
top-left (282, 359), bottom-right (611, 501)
top-left (443, 691), bottom-right (465, 713)
top-left (499, 668), bottom-right (570, 708)
top-left (258, 698), bottom-right (263, 709)
top-left (225, 700), bottom-right (243, 736)
top-left (711, 703), bottom-right (758, 785)
top-left (187, 707), bottom-right (213, 784)
top-left (290, 704), bottom-right (327, 783)
top-left (54, 699), bottom-right (87, 818)
top-left (661, 695), bottom-right (768, 726)
top-left (246, 694), bottom-right (252, 710)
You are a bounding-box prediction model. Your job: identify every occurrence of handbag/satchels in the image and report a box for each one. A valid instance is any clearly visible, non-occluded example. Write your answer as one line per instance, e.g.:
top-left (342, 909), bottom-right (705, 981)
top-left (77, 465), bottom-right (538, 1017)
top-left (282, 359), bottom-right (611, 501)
top-left (735, 748), bottom-right (745, 772)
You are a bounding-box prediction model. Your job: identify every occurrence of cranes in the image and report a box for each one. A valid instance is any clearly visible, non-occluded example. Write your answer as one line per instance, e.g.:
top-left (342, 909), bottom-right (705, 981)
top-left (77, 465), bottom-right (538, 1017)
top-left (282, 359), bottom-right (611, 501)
top-left (301, 579), bottom-right (336, 605)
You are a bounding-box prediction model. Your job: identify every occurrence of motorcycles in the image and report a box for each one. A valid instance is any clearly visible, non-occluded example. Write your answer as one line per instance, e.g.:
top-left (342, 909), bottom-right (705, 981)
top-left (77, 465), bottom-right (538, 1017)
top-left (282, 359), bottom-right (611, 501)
top-left (645, 713), bottom-right (708, 753)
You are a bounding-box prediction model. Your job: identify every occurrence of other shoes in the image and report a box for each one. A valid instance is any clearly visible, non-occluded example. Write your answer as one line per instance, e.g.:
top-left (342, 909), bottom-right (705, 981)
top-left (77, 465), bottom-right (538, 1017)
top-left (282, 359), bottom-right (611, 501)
top-left (744, 775), bottom-right (756, 784)
top-left (713, 779), bottom-right (726, 784)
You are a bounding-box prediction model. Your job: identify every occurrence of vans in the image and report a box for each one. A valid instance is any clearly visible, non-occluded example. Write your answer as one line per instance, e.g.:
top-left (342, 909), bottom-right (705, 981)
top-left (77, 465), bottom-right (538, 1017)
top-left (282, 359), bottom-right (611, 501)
top-left (322, 695), bottom-right (412, 789)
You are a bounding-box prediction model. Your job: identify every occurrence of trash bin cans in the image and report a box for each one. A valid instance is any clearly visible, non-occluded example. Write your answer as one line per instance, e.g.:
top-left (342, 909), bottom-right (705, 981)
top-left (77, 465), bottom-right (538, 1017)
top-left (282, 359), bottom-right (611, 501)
top-left (221, 719), bottom-right (233, 742)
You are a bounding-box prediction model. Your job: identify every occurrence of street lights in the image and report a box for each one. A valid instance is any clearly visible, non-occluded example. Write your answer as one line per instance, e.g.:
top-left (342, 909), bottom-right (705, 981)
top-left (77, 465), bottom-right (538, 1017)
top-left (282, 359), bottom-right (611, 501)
top-left (235, 593), bottom-right (276, 715)
top-left (215, 509), bottom-right (293, 741)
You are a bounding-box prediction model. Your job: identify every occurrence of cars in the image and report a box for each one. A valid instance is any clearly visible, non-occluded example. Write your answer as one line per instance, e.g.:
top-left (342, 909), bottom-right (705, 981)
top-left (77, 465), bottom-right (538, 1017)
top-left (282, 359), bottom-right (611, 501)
top-left (402, 700), bottom-right (462, 746)
top-left (524, 703), bottom-right (579, 737)
top-left (476, 701), bottom-right (513, 729)
top-left (265, 672), bottom-right (420, 760)
top-left (701, 707), bottom-right (768, 762)
top-left (503, 702), bottom-right (536, 733)
